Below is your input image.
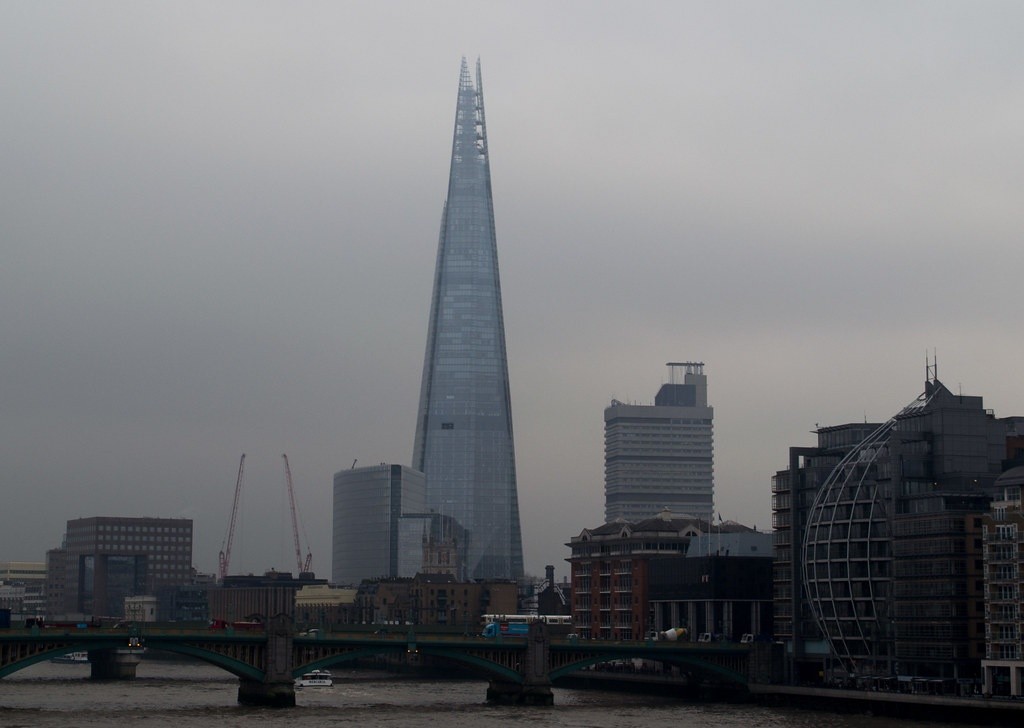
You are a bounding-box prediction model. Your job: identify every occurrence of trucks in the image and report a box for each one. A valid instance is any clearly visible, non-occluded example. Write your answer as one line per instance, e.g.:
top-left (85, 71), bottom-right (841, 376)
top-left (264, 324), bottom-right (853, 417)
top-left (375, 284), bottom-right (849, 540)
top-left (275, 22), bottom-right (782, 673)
top-left (740, 633), bottom-right (785, 644)
top-left (208, 619), bottom-right (263, 636)
top-left (698, 630), bottom-right (731, 643)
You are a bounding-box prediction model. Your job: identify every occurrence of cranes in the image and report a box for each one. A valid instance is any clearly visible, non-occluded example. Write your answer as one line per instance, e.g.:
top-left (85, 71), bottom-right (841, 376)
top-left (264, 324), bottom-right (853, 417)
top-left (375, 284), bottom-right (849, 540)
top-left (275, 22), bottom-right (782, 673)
top-left (215, 453), bottom-right (247, 585)
top-left (281, 453), bottom-right (314, 580)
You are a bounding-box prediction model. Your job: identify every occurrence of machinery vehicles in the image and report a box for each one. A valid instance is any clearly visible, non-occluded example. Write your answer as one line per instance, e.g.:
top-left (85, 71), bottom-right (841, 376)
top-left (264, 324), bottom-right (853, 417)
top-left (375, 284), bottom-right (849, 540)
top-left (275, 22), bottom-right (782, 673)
top-left (644, 627), bottom-right (688, 641)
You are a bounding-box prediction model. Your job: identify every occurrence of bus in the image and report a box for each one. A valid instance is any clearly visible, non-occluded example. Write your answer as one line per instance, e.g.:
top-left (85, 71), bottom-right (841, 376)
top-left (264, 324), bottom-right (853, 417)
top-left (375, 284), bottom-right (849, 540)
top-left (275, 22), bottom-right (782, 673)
top-left (91, 615), bottom-right (127, 633)
top-left (479, 614), bottom-right (574, 639)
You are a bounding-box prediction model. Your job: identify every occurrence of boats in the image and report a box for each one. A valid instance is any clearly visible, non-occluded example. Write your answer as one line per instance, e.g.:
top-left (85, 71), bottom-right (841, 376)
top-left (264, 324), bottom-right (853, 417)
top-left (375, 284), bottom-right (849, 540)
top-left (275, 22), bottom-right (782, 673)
top-left (295, 669), bottom-right (333, 687)
top-left (52, 651), bottom-right (87, 664)
